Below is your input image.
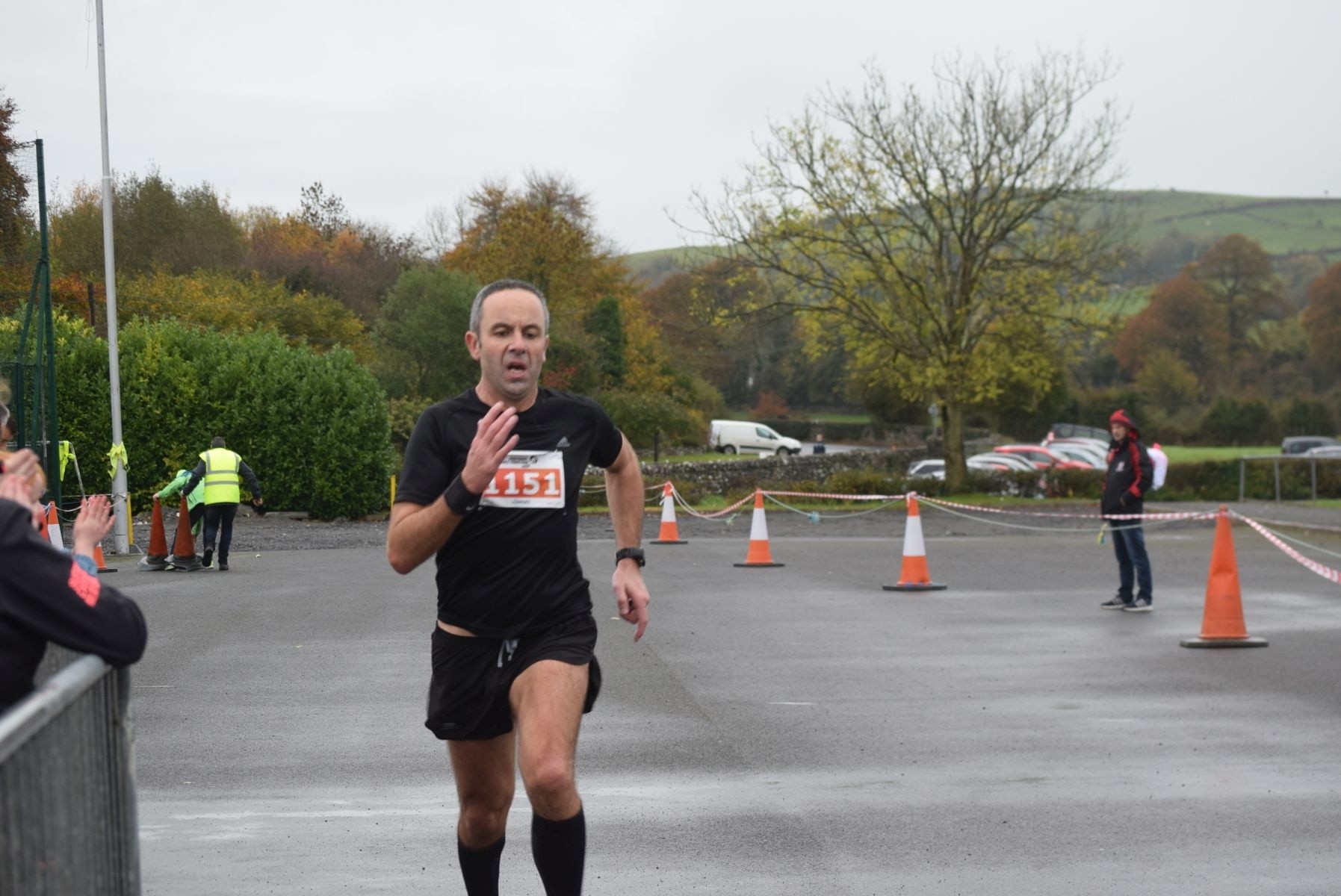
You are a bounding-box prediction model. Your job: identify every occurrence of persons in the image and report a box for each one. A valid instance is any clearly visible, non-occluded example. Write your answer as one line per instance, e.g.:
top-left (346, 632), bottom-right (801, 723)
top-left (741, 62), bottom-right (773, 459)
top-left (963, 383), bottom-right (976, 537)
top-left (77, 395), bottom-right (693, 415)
top-left (1101, 408), bottom-right (1155, 611)
top-left (180, 436), bottom-right (263, 571)
top-left (152, 469), bottom-right (205, 569)
top-left (385, 280), bottom-right (650, 896)
top-left (1, 375), bottom-right (148, 720)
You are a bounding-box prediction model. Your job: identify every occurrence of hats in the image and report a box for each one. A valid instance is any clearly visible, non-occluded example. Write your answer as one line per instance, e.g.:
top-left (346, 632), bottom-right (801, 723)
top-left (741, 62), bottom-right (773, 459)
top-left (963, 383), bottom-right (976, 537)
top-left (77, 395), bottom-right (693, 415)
top-left (176, 470), bottom-right (186, 477)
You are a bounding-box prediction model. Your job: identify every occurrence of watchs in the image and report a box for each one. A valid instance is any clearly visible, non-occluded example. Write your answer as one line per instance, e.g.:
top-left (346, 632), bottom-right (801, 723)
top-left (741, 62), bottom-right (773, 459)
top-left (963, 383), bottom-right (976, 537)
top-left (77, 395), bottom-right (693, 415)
top-left (616, 547), bottom-right (646, 567)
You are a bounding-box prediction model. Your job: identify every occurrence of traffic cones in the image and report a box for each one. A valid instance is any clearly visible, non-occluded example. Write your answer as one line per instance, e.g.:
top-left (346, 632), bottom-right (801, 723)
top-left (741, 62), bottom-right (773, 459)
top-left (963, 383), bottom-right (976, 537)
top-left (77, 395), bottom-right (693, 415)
top-left (647, 480), bottom-right (689, 545)
top-left (92, 540), bottom-right (118, 573)
top-left (732, 487), bottom-right (786, 568)
top-left (164, 495), bottom-right (204, 572)
top-left (1179, 505), bottom-right (1270, 649)
top-left (881, 491), bottom-right (949, 592)
top-left (138, 496), bottom-right (171, 571)
top-left (46, 500), bottom-right (65, 551)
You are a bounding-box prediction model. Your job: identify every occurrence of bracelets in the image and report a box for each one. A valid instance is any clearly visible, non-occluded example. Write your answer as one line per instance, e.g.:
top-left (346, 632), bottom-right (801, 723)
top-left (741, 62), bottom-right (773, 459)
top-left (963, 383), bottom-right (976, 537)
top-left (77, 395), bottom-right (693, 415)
top-left (443, 472), bottom-right (482, 516)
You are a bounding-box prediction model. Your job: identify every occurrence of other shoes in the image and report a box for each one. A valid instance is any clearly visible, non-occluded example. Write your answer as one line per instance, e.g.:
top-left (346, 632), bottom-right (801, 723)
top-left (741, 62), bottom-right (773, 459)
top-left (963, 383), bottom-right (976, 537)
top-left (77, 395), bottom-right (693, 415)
top-left (165, 562), bottom-right (174, 570)
top-left (205, 561), bottom-right (214, 569)
top-left (202, 547), bottom-right (213, 567)
top-left (220, 564), bottom-right (228, 570)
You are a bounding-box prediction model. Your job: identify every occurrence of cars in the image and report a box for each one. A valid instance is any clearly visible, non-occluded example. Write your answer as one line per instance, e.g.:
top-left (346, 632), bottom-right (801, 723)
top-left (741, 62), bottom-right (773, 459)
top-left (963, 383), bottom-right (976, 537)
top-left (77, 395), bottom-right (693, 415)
top-left (707, 418), bottom-right (803, 458)
top-left (1280, 434), bottom-right (1341, 455)
top-left (904, 421), bottom-right (1169, 501)
top-left (1305, 445), bottom-right (1341, 459)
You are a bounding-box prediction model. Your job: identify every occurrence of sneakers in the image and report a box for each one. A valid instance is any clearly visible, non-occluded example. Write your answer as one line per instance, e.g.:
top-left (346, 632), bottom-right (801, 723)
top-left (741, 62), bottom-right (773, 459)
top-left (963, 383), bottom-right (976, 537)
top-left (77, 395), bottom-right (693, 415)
top-left (1122, 595), bottom-right (1152, 611)
top-left (1101, 594), bottom-right (1134, 609)
